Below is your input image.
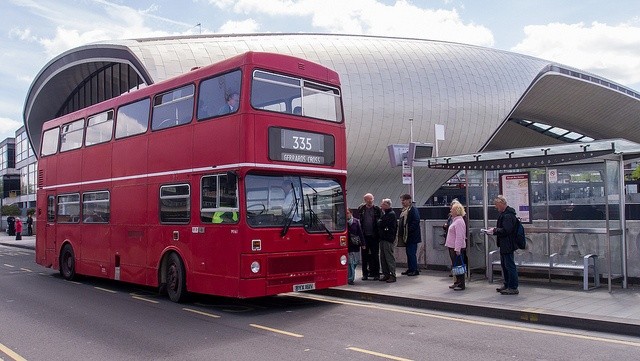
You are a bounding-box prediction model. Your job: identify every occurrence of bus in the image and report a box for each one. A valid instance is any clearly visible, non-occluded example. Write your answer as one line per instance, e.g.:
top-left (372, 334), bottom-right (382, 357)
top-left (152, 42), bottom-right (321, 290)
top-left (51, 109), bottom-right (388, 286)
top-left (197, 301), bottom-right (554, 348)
top-left (35, 50), bottom-right (349, 304)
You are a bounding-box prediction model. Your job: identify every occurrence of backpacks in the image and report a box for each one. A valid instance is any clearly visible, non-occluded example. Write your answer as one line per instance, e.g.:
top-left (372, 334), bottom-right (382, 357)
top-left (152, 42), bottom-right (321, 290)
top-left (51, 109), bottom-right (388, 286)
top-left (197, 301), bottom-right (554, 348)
top-left (504, 212), bottom-right (526, 249)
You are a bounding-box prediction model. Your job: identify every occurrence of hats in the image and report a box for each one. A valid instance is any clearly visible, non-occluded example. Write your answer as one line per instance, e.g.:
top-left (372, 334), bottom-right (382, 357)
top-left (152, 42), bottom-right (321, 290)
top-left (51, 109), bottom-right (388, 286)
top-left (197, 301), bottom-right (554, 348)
top-left (400, 194), bottom-right (412, 199)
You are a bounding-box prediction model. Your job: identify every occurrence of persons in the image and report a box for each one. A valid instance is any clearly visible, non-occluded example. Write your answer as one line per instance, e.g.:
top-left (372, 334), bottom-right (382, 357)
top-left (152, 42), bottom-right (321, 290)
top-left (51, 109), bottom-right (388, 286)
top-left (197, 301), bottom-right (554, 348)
top-left (15, 218), bottom-right (22, 240)
top-left (443, 198), bottom-right (472, 278)
top-left (281, 182), bottom-right (302, 222)
top-left (26, 215), bottom-right (34, 236)
top-left (218, 93), bottom-right (240, 116)
top-left (487, 194), bottom-right (525, 295)
top-left (377, 198), bottom-right (398, 283)
top-left (293, 106), bottom-right (304, 116)
top-left (445, 203), bottom-right (467, 290)
top-left (396, 193), bottom-right (422, 276)
top-left (347, 207), bottom-right (366, 285)
top-left (356, 193), bottom-right (384, 280)
top-left (7, 214), bottom-right (16, 236)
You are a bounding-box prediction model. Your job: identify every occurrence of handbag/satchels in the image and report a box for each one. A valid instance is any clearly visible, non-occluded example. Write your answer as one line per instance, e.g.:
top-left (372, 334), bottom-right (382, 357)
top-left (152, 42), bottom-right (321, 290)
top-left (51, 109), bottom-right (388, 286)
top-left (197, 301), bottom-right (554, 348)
top-left (452, 255), bottom-right (467, 275)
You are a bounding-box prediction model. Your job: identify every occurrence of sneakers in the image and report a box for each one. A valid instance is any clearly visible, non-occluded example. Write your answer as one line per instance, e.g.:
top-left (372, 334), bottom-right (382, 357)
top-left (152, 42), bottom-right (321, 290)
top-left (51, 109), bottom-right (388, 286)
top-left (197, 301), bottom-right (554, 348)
top-left (496, 285), bottom-right (507, 292)
top-left (500, 287), bottom-right (519, 295)
top-left (449, 285), bottom-right (455, 288)
top-left (454, 287), bottom-right (463, 290)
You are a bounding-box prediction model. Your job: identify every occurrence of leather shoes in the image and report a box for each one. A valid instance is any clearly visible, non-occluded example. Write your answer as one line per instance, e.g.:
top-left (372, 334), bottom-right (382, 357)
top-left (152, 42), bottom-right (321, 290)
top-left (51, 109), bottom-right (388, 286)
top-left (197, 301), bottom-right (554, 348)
top-left (401, 271), bottom-right (410, 274)
top-left (374, 276), bottom-right (379, 280)
top-left (379, 276), bottom-right (388, 281)
top-left (386, 278), bottom-right (396, 282)
top-left (362, 276), bottom-right (367, 279)
top-left (407, 271), bottom-right (419, 276)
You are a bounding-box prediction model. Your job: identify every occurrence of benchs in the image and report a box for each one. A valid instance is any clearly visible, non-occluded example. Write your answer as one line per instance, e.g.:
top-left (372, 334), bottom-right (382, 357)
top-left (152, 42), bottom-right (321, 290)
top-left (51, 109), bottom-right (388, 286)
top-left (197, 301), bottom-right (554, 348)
top-left (486, 250), bottom-right (601, 292)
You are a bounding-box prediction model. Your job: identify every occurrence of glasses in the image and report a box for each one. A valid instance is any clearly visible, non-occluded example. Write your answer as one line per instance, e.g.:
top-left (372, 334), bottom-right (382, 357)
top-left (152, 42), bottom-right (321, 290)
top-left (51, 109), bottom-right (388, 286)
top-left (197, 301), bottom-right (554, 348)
top-left (496, 197), bottom-right (504, 202)
